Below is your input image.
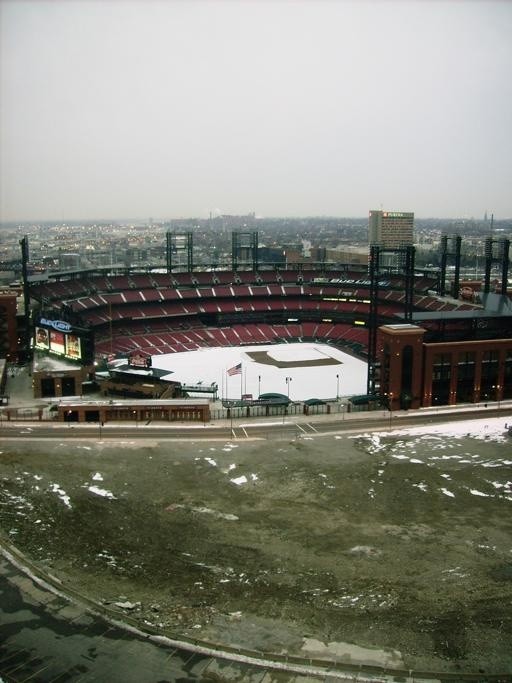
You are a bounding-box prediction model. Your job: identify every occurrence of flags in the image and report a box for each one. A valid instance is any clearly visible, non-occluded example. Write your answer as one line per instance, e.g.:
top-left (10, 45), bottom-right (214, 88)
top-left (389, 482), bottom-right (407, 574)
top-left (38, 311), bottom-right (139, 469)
top-left (226, 363), bottom-right (242, 376)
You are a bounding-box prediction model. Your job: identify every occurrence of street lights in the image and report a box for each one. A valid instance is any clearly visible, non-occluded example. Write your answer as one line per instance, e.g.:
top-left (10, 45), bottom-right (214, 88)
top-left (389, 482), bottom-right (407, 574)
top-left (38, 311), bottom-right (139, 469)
top-left (258, 375), bottom-right (261, 399)
top-left (336, 373), bottom-right (339, 400)
top-left (384, 391), bottom-right (394, 430)
top-left (492, 384), bottom-right (503, 416)
top-left (286, 376), bottom-right (292, 401)
